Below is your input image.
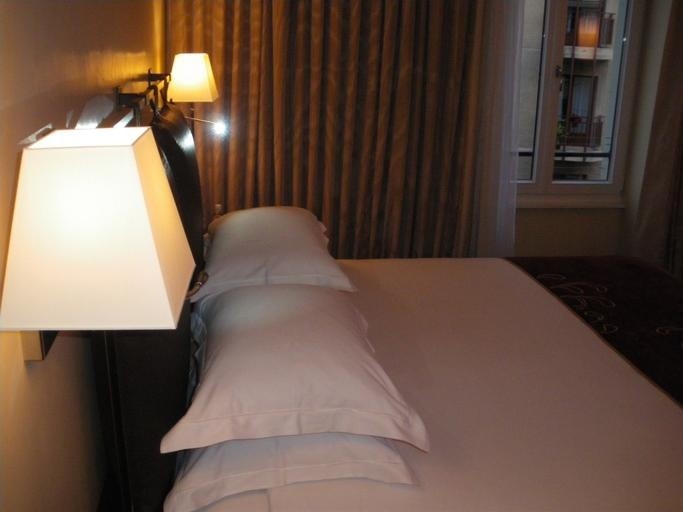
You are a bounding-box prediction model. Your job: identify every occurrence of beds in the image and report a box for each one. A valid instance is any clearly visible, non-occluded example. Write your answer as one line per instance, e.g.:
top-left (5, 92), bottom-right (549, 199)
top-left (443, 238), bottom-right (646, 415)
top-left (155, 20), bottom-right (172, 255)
top-left (106, 119), bottom-right (683, 511)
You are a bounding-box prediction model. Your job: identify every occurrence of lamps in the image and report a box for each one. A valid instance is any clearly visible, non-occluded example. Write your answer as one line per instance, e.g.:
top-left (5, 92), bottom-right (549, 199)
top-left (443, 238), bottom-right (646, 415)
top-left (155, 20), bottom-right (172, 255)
top-left (164, 47), bottom-right (220, 110)
top-left (0, 122), bottom-right (197, 341)
top-left (184, 113), bottom-right (229, 135)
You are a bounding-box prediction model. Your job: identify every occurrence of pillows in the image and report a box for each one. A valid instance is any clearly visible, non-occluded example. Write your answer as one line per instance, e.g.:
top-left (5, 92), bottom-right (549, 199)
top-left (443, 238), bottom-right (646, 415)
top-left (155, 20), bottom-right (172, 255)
top-left (161, 291), bottom-right (431, 455)
top-left (162, 434), bottom-right (413, 512)
top-left (190, 205), bottom-right (353, 300)
